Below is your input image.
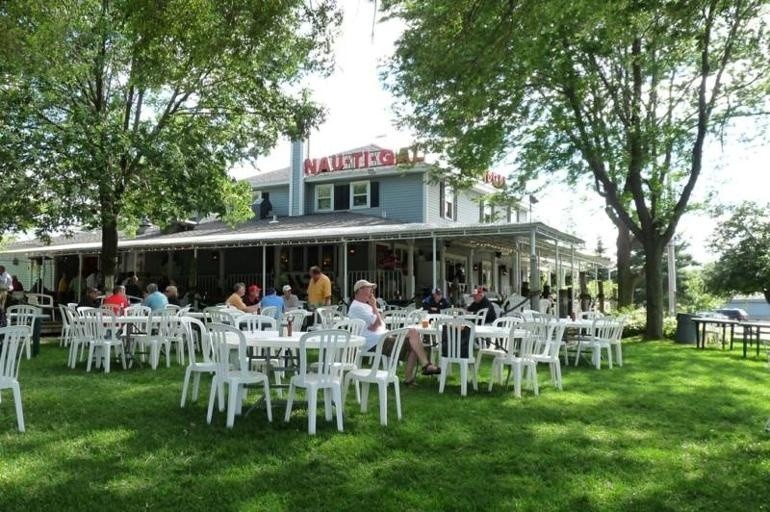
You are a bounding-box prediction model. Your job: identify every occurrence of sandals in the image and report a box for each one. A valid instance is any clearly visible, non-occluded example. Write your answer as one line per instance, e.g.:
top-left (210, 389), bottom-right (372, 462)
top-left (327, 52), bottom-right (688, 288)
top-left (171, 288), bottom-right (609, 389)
top-left (421, 363), bottom-right (441, 375)
top-left (403, 379), bottom-right (423, 391)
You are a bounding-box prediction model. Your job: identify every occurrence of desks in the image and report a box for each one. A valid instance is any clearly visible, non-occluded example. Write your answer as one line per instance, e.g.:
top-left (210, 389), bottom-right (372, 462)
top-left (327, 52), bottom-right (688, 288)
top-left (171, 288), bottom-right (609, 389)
top-left (691, 317), bottom-right (770, 358)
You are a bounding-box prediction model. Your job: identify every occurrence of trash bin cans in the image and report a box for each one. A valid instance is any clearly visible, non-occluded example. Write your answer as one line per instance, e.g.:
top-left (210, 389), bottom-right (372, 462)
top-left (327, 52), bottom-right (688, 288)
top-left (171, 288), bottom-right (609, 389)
top-left (4, 303), bottom-right (40, 358)
top-left (675, 313), bottom-right (701, 343)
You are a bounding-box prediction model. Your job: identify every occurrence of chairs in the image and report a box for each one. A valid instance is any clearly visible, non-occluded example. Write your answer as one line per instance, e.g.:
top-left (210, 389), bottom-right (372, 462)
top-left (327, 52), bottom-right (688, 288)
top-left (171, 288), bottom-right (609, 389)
top-left (0, 294), bottom-right (630, 435)
top-left (292, 272), bottom-right (310, 294)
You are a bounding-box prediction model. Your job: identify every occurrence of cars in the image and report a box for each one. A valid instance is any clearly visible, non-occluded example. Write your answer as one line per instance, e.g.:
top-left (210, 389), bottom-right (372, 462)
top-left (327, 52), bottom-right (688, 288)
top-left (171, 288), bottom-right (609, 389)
top-left (713, 307), bottom-right (750, 322)
top-left (698, 312), bottom-right (728, 320)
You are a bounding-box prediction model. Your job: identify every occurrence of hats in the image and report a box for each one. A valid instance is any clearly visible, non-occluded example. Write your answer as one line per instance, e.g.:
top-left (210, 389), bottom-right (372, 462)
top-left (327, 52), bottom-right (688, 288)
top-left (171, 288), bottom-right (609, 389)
top-left (469, 289), bottom-right (482, 298)
top-left (247, 285), bottom-right (262, 293)
top-left (282, 285), bottom-right (292, 292)
top-left (431, 288), bottom-right (443, 296)
top-left (353, 279), bottom-right (378, 293)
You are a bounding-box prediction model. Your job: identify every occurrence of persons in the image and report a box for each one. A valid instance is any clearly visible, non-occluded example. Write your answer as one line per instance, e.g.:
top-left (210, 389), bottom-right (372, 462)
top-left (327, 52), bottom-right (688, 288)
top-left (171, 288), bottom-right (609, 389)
top-left (305, 265), bottom-right (332, 309)
top-left (347, 279), bottom-right (440, 385)
top-left (260, 287), bottom-right (286, 318)
top-left (246, 286), bottom-right (262, 306)
top-left (282, 284), bottom-right (298, 311)
top-left (0, 263), bottom-right (180, 328)
top-left (421, 288), bottom-right (450, 313)
top-left (522, 281), bottom-right (531, 297)
top-left (543, 284), bottom-right (554, 298)
top-left (225, 283), bottom-right (260, 313)
top-left (461, 287), bottom-right (498, 351)
